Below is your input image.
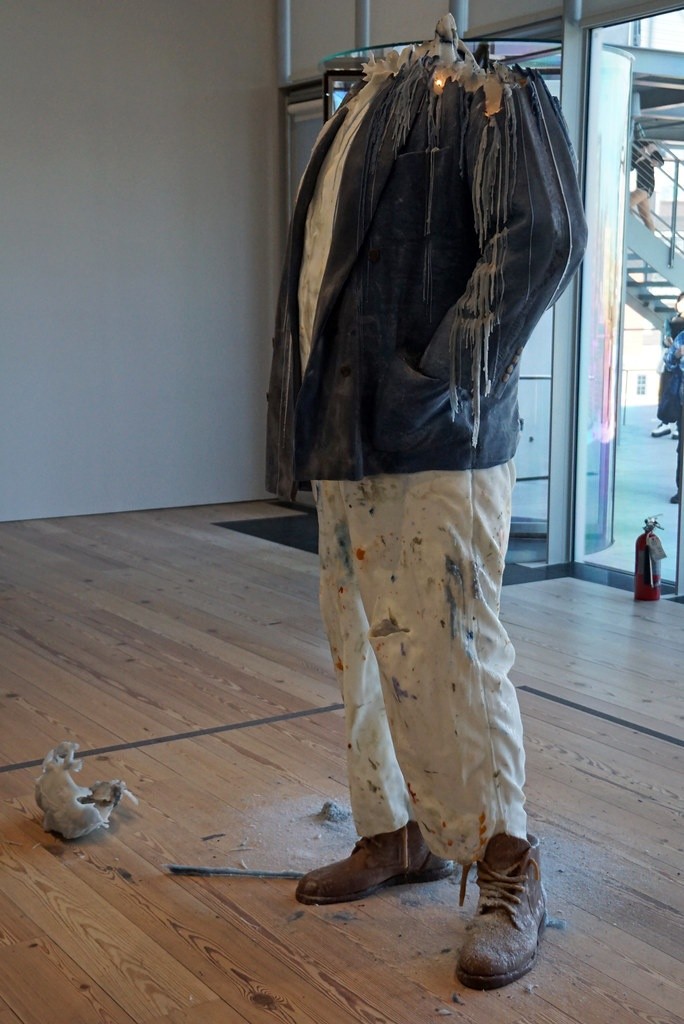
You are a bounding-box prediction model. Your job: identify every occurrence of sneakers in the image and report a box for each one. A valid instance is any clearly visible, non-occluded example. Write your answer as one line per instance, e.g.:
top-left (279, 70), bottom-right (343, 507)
top-left (294, 816), bottom-right (456, 902)
top-left (458, 830), bottom-right (549, 986)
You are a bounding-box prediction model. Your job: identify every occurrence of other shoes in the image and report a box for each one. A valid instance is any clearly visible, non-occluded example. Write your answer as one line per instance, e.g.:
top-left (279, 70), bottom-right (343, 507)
top-left (652, 423), bottom-right (671, 437)
top-left (671, 430), bottom-right (678, 440)
top-left (670, 494), bottom-right (679, 504)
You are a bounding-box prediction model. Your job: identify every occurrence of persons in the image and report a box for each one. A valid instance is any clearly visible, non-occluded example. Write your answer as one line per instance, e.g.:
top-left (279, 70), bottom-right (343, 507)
top-left (262, 14), bottom-right (591, 991)
top-left (632, 137), bottom-right (665, 233)
top-left (651, 293), bottom-right (684, 504)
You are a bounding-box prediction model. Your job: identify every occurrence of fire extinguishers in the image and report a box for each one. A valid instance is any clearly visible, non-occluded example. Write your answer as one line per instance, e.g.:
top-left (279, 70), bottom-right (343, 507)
top-left (634, 513), bottom-right (665, 601)
